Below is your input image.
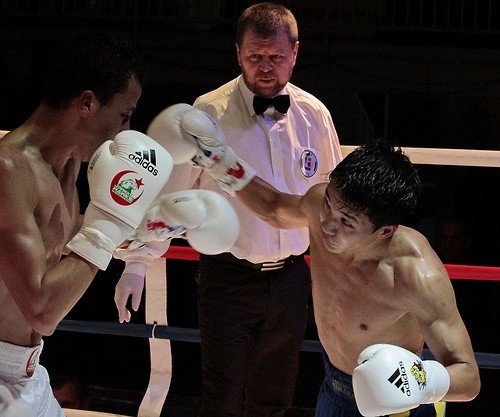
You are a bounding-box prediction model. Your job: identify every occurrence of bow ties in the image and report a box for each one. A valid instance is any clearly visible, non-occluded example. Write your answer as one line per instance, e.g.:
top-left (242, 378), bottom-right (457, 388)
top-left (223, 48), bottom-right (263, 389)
top-left (252, 95), bottom-right (290, 116)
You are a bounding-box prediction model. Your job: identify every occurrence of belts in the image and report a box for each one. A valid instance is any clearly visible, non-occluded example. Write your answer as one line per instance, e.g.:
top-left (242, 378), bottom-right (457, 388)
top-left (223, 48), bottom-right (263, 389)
top-left (200, 254), bottom-right (304, 272)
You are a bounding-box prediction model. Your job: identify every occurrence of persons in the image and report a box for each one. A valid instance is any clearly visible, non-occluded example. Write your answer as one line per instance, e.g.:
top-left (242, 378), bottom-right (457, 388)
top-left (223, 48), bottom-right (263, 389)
top-left (114, 3), bottom-right (343, 417)
top-left (147, 103), bottom-right (481, 417)
top-left (0, 41), bottom-right (241, 417)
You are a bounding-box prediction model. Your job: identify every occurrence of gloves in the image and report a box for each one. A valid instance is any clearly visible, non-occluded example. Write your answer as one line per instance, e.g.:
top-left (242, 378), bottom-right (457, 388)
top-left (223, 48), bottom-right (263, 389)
top-left (65, 130), bottom-right (173, 271)
top-left (147, 103), bottom-right (256, 199)
top-left (352, 344), bottom-right (451, 417)
top-left (137, 190), bottom-right (240, 255)
